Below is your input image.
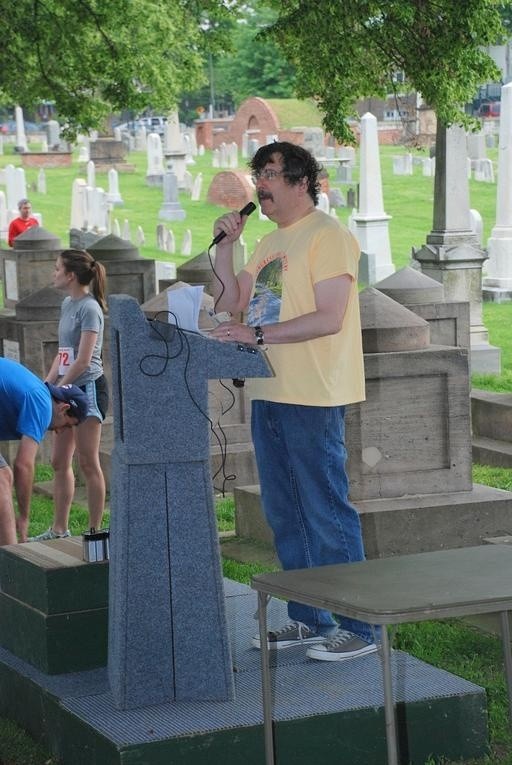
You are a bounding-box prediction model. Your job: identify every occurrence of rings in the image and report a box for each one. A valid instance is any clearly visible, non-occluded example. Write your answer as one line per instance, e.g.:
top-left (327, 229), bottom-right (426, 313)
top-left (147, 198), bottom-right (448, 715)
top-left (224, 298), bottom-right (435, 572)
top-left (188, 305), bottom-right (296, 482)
top-left (227, 329), bottom-right (230, 336)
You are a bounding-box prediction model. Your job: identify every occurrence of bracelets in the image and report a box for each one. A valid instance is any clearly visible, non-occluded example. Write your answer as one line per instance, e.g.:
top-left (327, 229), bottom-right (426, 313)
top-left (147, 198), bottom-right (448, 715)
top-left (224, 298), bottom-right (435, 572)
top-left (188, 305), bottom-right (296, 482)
top-left (255, 324), bottom-right (265, 344)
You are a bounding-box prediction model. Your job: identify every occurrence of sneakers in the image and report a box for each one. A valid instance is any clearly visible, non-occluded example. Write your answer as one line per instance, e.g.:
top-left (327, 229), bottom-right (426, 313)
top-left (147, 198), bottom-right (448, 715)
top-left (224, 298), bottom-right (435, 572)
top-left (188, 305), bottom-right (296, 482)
top-left (305, 629), bottom-right (383, 662)
top-left (250, 620), bottom-right (327, 650)
top-left (27, 527), bottom-right (71, 542)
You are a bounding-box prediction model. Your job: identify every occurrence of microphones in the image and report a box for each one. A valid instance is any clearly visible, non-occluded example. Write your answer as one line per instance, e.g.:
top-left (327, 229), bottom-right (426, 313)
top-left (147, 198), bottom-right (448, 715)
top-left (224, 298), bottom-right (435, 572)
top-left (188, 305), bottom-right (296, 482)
top-left (212, 201), bottom-right (256, 244)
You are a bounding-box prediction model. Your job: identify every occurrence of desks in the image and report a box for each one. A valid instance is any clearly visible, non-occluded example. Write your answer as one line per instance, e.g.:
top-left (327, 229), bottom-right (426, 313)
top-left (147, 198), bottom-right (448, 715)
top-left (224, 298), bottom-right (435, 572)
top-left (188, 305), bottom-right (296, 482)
top-left (251, 543), bottom-right (512, 765)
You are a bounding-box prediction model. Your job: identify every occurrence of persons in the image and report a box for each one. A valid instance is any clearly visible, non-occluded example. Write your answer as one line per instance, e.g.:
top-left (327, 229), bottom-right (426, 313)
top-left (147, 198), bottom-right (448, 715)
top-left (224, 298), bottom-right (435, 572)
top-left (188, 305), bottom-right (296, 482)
top-left (8, 198), bottom-right (41, 248)
top-left (209, 139), bottom-right (379, 661)
top-left (23, 248), bottom-right (108, 543)
top-left (0, 356), bottom-right (87, 543)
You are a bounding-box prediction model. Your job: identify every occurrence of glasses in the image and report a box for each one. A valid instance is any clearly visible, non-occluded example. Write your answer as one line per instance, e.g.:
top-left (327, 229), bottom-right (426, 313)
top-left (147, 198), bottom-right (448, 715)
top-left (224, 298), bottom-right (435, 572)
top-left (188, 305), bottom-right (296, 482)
top-left (251, 170), bottom-right (280, 185)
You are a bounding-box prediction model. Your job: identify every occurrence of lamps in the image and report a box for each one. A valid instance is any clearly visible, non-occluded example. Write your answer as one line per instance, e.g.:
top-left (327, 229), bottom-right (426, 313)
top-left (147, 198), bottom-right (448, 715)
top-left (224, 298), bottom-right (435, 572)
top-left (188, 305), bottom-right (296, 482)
top-left (82, 527), bottom-right (107, 562)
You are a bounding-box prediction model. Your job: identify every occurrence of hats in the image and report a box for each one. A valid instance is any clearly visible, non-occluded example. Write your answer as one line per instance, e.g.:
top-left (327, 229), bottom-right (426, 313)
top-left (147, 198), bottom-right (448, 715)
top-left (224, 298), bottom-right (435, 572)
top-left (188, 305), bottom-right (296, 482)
top-left (44, 381), bottom-right (89, 423)
